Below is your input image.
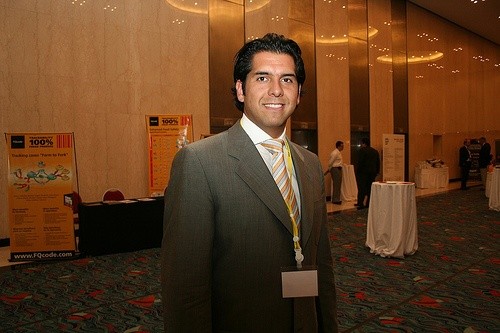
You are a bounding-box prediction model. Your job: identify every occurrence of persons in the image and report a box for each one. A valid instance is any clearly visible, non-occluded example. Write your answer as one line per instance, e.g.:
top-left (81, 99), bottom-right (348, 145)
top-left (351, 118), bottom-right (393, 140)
top-left (162, 34), bottom-right (337, 333)
top-left (478, 137), bottom-right (493, 191)
top-left (354, 137), bottom-right (380, 206)
top-left (457, 139), bottom-right (473, 189)
top-left (328, 141), bottom-right (344, 204)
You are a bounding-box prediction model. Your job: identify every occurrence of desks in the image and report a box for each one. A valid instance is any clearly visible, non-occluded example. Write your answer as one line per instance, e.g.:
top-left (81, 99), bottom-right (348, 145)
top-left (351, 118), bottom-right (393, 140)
top-left (330, 165), bottom-right (358, 202)
top-left (415, 168), bottom-right (449, 189)
top-left (365, 182), bottom-right (418, 259)
top-left (78, 196), bottom-right (165, 258)
top-left (488, 168), bottom-right (500, 211)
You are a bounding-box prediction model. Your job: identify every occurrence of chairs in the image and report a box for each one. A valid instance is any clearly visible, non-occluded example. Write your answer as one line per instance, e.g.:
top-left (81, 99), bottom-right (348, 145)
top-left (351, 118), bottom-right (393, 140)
top-left (102, 188), bottom-right (124, 202)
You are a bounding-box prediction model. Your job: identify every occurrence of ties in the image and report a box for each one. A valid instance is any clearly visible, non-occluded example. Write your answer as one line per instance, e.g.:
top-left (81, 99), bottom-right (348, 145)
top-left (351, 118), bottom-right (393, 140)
top-left (261, 139), bottom-right (301, 242)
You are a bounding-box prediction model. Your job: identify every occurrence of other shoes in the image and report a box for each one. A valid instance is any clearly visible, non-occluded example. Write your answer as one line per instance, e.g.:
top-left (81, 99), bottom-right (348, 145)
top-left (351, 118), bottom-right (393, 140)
top-left (357, 206), bottom-right (369, 210)
top-left (332, 200), bottom-right (343, 205)
top-left (354, 204), bottom-right (363, 207)
